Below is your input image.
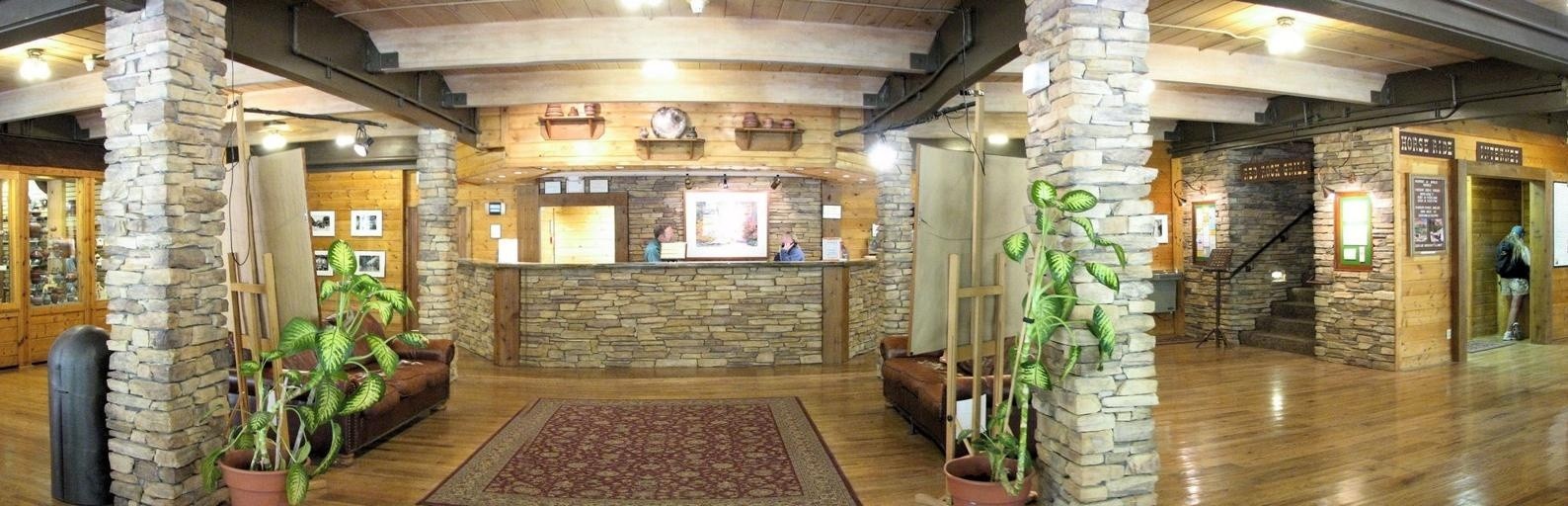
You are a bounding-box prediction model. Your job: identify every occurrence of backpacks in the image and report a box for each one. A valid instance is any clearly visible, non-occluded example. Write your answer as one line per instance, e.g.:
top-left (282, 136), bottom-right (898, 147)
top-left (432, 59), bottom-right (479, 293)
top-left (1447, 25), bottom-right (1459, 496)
top-left (1494, 241), bottom-right (1513, 273)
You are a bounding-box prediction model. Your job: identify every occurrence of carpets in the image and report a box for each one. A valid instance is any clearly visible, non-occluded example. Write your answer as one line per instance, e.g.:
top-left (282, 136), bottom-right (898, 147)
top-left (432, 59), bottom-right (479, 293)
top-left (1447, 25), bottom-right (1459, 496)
top-left (415, 396), bottom-right (864, 506)
top-left (1467, 340), bottom-right (1516, 352)
top-left (1156, 335), bottom-right (1208, 345)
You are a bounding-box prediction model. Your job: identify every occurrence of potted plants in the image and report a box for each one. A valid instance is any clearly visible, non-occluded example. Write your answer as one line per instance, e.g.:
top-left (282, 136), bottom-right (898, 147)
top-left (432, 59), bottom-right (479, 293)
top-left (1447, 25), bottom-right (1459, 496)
top-left (199, 240), bottom-right (430, 506)
top-left (943, 179), bottom-right (1126, 506)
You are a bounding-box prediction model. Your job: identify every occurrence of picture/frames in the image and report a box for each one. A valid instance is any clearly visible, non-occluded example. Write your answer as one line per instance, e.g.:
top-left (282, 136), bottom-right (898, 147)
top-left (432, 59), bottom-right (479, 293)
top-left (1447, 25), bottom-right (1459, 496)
top-left (313, 249), bottom-right (335, 277)
top-left (309, 209), bottom-right (336, 238)
top-left (353, 250), bottom-right (386, 279)
top-left (1552, 181), bottom-right (1568, 268)
top-left (350, 209), bottom-right (383, 238)
top-left (683, 189), bottom-right (769, 260)
top-left (1405, 173), bottom-right (1449, 257)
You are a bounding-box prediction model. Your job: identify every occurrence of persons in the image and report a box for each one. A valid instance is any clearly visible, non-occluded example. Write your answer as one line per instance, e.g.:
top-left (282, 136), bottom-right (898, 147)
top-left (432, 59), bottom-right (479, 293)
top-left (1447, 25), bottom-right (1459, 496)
top-left (772, 231), bottom-right (805, 261)
top-left (644, 225), bottom-right (678, 262)
top-left (1494, 225), bottom-right (1531, 340)
top-left (33, 240), bottom-right (78, 301)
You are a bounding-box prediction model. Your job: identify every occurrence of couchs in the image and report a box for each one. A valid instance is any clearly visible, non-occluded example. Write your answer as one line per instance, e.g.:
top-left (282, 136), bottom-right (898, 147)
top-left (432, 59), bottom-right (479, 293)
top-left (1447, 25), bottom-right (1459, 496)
top-left (227, 308), bottom-right (456, 466)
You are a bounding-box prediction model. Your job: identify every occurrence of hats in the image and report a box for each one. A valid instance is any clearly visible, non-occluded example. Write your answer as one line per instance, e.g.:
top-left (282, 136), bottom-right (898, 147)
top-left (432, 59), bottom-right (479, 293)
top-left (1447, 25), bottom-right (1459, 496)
top-left (1511, 226), bottom-right (1526, 235)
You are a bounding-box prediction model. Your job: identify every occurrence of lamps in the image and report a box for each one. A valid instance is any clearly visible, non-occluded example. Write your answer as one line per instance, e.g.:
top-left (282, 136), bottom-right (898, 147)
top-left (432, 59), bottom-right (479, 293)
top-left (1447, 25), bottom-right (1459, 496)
top-left (685, 173), bottom-right (693, 189)
top-left (260, 121), bottom-right (289, 152)
top-left (1265, 17), bottom-right (1306, 56)
top-left (771, 175), bottom-right (781, 189)
top-left (722, 174), bottom-right (728, 189)
top-left (18, 48), bottom-right (51, 80)
top-left (243, 108), bottom-right (387, 158)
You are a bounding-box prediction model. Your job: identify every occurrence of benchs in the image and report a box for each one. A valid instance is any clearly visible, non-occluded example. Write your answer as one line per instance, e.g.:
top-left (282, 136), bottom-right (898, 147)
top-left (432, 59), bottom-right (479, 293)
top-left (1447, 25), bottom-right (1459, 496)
top-left (880, 335), bottom-right (1039, 460)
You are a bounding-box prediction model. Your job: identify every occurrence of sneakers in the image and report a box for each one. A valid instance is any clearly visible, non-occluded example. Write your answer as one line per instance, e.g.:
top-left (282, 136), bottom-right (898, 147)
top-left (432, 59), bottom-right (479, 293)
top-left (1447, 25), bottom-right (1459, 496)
top-left (1502, 332), bottom-right (1517, 340)
top-left (1512, 322), bottom-right (1521, 339)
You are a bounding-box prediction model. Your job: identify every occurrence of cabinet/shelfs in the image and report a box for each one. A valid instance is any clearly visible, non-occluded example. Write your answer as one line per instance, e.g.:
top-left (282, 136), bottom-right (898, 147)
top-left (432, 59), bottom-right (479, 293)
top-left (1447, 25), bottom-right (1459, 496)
top-left (0, 165), bottom-right (111, 368)
top-left (539, 116), bottom-right (799, 160)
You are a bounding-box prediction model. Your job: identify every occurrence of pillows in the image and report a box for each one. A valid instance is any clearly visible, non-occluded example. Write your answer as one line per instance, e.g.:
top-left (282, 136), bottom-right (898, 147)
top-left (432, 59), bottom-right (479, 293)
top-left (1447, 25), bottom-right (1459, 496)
top-left (941, 340), bottom-right (995, 375)
top-left (322, 309), bottom-right (390, 368)
